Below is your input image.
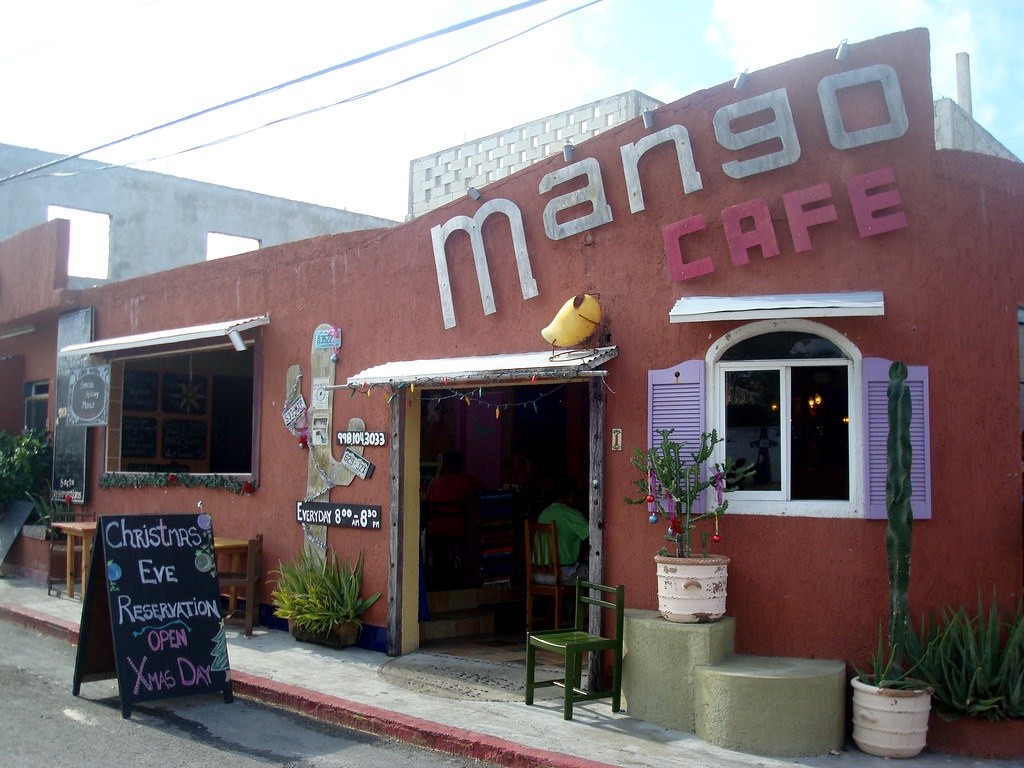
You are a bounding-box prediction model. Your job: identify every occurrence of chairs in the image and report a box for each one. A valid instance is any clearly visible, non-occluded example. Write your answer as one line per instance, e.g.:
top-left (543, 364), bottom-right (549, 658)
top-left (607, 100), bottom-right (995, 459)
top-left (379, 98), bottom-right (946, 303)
top-left (47, 510), bottom-right (95, 597)
top-left (422, 500), bottom-right (469, 573)
top-left (524, 520), bottom-right (576, 633)
top-left (525, 576), bottom-right (624, 720)
top-left (217, 534), bottom-right (263, 636)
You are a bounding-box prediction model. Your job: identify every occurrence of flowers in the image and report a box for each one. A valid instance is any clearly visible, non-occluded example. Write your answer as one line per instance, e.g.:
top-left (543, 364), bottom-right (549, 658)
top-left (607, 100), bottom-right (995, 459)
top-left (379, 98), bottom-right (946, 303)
top-left (97, 471), bottom-right (258, 496)
top-left (23, 478), bottom-right (72, 538)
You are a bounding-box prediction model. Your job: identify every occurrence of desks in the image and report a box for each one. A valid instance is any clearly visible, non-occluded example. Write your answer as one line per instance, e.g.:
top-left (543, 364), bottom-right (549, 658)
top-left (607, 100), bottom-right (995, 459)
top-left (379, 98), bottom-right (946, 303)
top-left (51, 522), bottom-right (97, 602)
top-left (214, 536), bottom-right (249, 626)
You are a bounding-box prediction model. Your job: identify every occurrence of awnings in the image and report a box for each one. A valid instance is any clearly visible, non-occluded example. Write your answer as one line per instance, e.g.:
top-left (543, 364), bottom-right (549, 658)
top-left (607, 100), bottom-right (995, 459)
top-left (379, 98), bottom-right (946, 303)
top-left (52, 314), bottom-right (272, 360)
top-left (667, 290), bottom-right (886, 325)
top-left (345, 343), bottom-right (619, 386)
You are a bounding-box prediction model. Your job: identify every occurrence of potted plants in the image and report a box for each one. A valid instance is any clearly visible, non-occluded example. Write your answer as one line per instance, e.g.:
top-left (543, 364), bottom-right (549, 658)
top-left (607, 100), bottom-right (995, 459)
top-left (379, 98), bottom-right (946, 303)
top-left (851, 360), bottom-right (944, 760)
top-left (902, 584), bottom-right (1024, 759)
top-left (623, 427), bottom-right (757, 624)
top-left (270, 543), bottom-right (382, 650)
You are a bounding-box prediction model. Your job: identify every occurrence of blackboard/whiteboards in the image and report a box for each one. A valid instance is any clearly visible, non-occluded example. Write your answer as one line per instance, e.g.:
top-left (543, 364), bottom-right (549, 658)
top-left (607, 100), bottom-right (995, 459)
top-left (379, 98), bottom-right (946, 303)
top-left (71, 513), bottom-right (234, 720)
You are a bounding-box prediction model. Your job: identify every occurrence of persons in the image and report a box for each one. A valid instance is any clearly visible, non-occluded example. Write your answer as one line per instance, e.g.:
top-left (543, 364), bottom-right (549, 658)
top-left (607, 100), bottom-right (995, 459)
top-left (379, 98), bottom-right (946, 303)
top-left (424, 451), bottom-right (534, 559)
top-left (529, 476), bottom-right (589, 629)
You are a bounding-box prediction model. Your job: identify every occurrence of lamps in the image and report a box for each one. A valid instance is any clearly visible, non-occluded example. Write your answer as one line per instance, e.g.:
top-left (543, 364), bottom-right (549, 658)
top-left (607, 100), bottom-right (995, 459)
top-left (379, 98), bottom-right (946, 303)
top-left (642, 107), bottom-right (654, 129)
top-left (466, 187), bottom-right (481, 201)
top-left (836, 38), bottom-right (848, 63)
top-left (732, 69), bottom-right (748, 90)
top-left (563, 140), bottom-right (574, 162)
